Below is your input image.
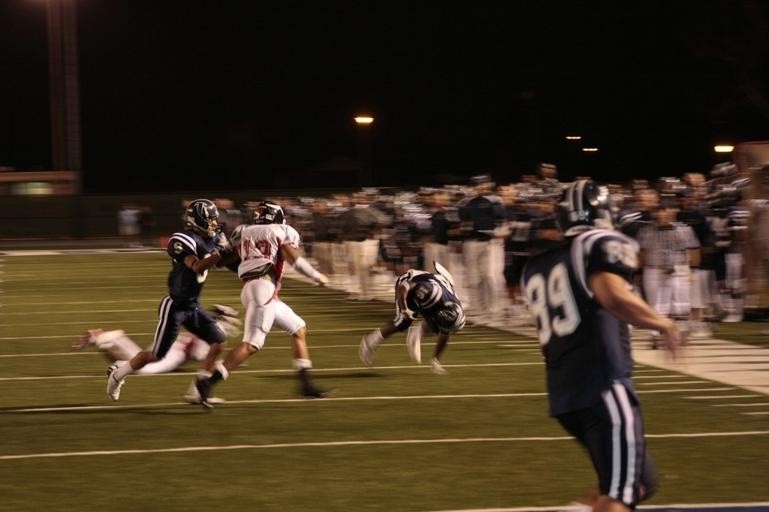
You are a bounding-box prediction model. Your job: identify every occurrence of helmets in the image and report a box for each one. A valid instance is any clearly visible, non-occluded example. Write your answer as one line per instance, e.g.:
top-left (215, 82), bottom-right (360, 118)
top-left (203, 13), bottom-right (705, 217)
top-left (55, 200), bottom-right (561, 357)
top-left (556, 177), bottom-right (613, 236)
top-left (433, 301), bottom-right (467, 334)
top-left (252, 200), bottom-right (286, 225)
top-left (205, 304), bottom-right (238, 337)
top-left (184, 198), bottom-right (220, 237)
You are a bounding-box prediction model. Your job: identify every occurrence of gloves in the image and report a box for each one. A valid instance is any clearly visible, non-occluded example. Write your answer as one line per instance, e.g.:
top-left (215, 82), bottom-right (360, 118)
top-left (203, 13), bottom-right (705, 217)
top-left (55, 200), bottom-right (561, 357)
top-left (314, 272), bottom-right (330, 287)
top-left (428, 355), bottom-right (449, 376)
top-left (214, 248), bottom-right (231, 266)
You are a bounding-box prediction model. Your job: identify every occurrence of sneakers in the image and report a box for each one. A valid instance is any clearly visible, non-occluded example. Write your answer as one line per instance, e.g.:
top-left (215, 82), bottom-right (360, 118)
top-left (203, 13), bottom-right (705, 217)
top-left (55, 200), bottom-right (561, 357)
top-left (406, 327), bottom-right (423, 364)
top-left (186, 376), bottom-right (225, 410)
top-left (70, 327), bottom-right (126, 402)
top-left (294, 369), bottom-right (339, 399)
top-left (359, 332), bottom-right (374, 366)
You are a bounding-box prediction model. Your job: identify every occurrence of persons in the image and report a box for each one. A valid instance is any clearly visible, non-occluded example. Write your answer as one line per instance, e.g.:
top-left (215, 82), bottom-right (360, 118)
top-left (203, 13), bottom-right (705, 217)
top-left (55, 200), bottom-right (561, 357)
top-left (519, 180), bottom-right (687, 512)
top-left (274, 165), bottom-right (571, 327)
top-left (119, 203), bottom-right (140, 248)
top-left (192, 199), bottom-right (341, 412)
top-left (104, 197), bottom-right (242, 405)
top-left (134, 204), bottom-right (159, 247)
top-left (357, 260), bottom-right (466, 375)
top-left (67, 305), bottom-right (240, 376)
top-left (610, 141), bottom-right (769, 352)
top-left (213, 197), bottom-right (242, 272)
